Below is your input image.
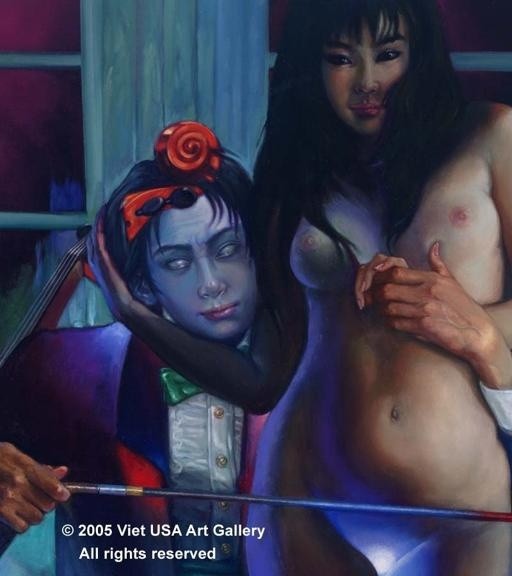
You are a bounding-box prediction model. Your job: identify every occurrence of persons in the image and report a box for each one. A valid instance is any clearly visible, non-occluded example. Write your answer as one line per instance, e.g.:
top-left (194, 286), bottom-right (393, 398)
top-left (1, 121), bottom-right (512, 576)
top-left (80, 0), bottom-right (510, 576)
top-left (0, 141), bottom-right (90, 576)
